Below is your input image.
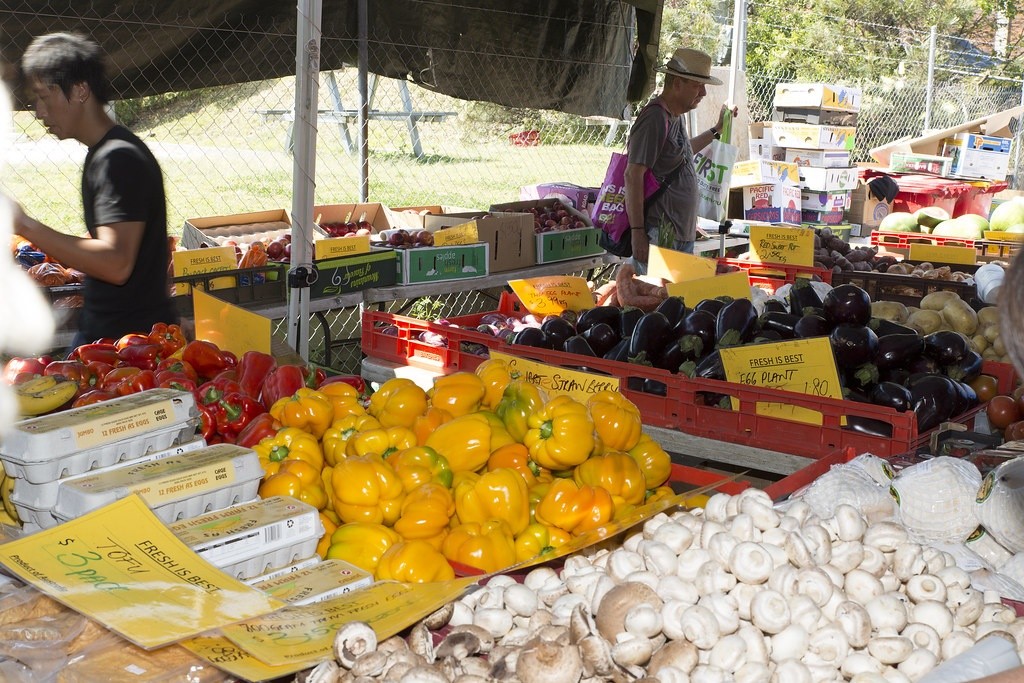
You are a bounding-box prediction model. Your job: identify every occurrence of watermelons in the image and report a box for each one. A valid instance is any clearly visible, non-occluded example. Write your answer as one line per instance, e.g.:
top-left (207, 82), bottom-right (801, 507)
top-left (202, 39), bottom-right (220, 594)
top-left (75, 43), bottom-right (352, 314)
top-left (881, 197), bottom-right (1024, 240)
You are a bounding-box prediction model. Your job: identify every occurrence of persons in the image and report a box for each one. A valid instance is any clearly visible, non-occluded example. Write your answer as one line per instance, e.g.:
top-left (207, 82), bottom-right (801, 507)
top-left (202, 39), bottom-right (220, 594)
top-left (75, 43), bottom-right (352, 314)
top-left (0, 31), bottom-right (170, 360)
top-left (623, 47), bottom-right (739, 275)
top-left (997, 244), bottom-right (1024, 384)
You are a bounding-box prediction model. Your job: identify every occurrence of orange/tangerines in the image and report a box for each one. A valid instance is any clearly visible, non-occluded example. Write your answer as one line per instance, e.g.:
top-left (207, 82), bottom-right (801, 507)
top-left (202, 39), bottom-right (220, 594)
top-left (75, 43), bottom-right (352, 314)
top-left (11, 234), bottom-right (181, 308)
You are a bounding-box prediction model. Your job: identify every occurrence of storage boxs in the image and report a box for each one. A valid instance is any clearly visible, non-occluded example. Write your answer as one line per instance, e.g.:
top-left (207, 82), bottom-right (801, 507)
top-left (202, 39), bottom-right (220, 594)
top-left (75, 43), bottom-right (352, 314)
top-left (390, 206), bottom-right (443, 218)
top-left (942, 132), bottom-right (1013, 183)
top-left (489, 197), bottom-right (608, 266)
top-left (0, 386), bottom-right (204, 483)
top-left (369, 238), bottom-right (491, 286)
top-left (889, 151), bottom-right (953, 178)
top-left (521, 182), bottom-right (589, 212)
top-left (891, 174), bottom-right (1008, 222)
top-left (166, 496), bottom-right (326, 580)
top-left (267, 250), bottom-right (397, 303)
top-left (424, 210), bottom-right (538, 272)
top-left (985, 107), bottom-right (1021, 136)
top-left (181, 208), bottom-right (293, 248)
top-left (729, 80), bottom-right (862, 242)
top-left (847, 183), bottom-right (895, 228)
top-left (313, 203), bottom-right (391, 243)
top-left (50, 443), bottom-right (267, 527)
top-left (248, 561), bottom-right (372, 606)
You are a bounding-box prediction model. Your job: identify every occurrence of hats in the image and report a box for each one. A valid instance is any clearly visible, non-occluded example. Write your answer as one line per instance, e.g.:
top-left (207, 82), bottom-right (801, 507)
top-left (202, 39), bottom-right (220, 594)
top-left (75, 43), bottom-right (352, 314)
top-left (653, 48), bottom-right (724, 85)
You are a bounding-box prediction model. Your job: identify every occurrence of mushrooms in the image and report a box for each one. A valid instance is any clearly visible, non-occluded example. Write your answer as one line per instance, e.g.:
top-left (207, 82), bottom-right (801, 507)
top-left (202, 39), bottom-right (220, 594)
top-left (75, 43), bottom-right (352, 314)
top-left (305, 487), bottom-right (1024, 682)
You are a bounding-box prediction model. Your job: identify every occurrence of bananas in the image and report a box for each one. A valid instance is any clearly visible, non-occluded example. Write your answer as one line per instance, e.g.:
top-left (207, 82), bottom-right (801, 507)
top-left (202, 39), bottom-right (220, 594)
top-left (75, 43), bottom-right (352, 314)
top-left (0, 376), bottom-right (80, 527)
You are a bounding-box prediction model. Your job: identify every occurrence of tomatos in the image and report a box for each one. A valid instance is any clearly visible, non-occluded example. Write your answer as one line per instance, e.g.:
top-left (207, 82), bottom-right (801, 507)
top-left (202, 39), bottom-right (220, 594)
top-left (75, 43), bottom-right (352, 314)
top-left (971, 375), bottom-right (1024, 441)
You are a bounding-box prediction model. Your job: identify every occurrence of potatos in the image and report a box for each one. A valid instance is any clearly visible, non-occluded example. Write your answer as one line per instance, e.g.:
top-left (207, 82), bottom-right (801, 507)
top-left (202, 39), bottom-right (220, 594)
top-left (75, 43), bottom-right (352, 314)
top-left (870, 290), bottom-right (1014, 366)
top-left (587, 263), bottom-right (672, 312)
top-left (808, 225), bottom-right (897, 273)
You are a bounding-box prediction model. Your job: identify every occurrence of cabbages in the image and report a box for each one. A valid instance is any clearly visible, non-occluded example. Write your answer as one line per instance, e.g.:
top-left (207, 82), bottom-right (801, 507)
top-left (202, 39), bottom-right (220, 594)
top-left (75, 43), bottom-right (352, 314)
top-left (773, 451), bottom-right (1024, 602)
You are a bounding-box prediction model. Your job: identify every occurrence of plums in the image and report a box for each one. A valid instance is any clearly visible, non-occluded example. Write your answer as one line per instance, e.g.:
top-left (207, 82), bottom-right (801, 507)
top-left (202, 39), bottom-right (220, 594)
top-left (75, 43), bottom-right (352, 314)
top-left (471, 201), bottom-right (586, 234)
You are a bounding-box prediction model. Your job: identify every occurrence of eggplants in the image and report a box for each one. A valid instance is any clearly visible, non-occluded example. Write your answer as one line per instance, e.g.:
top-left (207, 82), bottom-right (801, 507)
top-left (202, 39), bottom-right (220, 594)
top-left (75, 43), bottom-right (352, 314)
top-left (516, 278), bottom-right (980, 437)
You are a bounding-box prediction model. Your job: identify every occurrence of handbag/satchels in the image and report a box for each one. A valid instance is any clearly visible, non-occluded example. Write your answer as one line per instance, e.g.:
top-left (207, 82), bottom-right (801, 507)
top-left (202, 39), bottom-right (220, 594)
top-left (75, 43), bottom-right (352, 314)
top-left (591, 152), bottom-right (660, 243)
top-left (599, 221), bottom-right (654, 258)
top-left (692, 108), bottom-right (739, 222)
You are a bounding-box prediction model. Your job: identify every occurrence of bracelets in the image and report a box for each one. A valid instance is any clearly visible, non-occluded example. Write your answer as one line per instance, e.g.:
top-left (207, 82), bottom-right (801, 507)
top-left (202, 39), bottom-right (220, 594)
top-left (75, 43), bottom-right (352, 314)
top-left (710, 127), bottom-right (720, 140)
top-left (629, 227), bottom-right (645, 230)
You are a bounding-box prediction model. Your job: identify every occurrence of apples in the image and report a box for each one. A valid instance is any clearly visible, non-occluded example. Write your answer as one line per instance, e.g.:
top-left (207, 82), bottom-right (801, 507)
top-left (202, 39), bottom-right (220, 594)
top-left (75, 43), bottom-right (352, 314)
top-left (267, 221), bottom-right (432, 262)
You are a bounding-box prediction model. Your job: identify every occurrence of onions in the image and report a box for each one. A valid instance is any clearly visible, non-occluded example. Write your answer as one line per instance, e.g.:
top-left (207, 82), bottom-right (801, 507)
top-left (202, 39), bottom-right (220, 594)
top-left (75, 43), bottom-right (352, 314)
top-left (382, 309), bottom-right (587, 355)
top-left (887, 261), bottom-right (973, 281)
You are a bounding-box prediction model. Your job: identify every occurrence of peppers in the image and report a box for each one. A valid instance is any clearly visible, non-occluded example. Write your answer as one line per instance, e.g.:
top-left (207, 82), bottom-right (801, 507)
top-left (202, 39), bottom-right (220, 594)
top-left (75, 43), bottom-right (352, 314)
top-left (251, 358), bottom-right (711, 585)
top-left (7, 323), bottom-right (366, 448)
